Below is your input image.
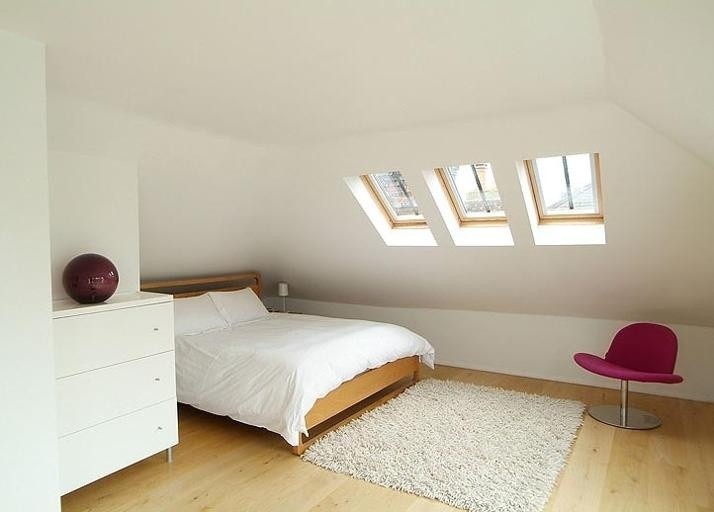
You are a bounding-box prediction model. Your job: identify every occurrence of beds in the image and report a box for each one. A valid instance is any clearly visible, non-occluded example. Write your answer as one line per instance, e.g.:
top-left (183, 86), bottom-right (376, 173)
top-left (141, 271), bottom-right (420, 457)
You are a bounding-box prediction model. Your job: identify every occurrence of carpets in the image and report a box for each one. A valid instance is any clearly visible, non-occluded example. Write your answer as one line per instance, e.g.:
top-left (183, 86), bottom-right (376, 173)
top-left (302, 378), bottom-right (588, 512)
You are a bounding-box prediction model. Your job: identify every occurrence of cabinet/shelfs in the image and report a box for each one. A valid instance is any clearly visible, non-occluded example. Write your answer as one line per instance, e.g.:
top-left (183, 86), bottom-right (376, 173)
top-left (573, 322), bottom-right (685, 430)
top-left (52, 292), bottom-right (180, 498)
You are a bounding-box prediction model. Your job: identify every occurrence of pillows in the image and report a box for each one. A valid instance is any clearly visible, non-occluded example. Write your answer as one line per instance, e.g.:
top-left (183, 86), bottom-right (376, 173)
top-left (174, 288), bottom-right (270, 337)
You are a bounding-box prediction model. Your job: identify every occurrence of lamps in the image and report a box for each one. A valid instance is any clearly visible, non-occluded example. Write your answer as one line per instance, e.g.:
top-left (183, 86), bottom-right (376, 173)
top-left (278, 281), bottom-right (289, 312)
